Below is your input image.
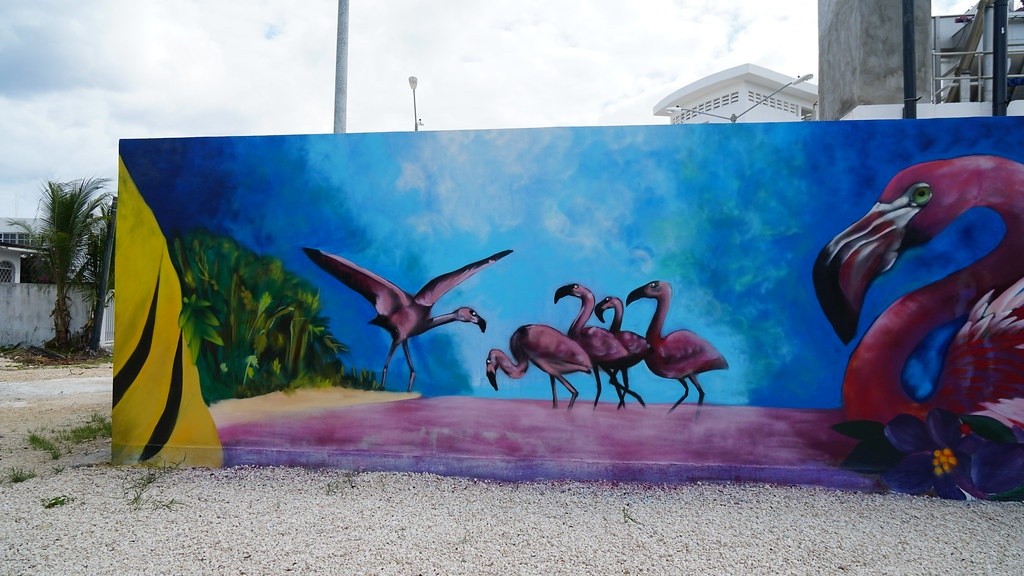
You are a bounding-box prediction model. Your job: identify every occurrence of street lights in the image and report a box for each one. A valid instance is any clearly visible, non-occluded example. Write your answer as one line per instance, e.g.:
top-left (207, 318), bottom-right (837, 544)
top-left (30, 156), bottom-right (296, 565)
top-left (665, 73), bottom-right (812, 123)
top-left (409, 76), bottom-right (420, 131)
